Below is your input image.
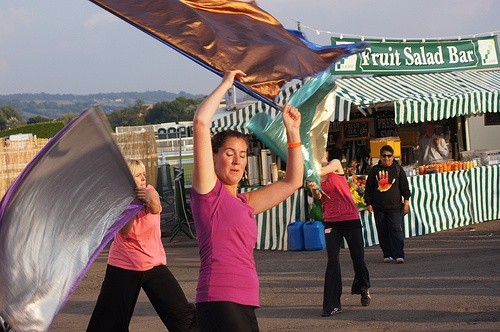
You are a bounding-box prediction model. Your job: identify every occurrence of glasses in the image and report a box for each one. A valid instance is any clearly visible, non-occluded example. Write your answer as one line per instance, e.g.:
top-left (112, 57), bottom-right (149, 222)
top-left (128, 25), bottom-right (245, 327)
top-left (381, 154), bottom-right (392, 158)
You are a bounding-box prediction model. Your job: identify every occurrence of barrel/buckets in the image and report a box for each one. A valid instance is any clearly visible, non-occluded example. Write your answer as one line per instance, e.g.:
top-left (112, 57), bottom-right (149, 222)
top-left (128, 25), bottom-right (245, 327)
top-left (303, 221), bottom-right (326, 251)
top-left (287, 221), bottom-right (306, 252)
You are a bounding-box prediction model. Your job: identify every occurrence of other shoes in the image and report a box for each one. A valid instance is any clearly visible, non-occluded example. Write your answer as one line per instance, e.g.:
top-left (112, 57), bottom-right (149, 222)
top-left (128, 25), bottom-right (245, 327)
top-left (360, 288), bottom-right (371, 305)
top-left (384, 258), bottom-right (391, 262)
top-left (396, 258), bottom-right (404, 263)
top-left (322, 307), bottom-right (343, 317)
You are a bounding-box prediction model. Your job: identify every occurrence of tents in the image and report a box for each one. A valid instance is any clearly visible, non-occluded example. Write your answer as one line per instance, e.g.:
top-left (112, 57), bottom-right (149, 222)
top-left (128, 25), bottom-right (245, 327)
top-left (209, 70), bottom-right (500, 250)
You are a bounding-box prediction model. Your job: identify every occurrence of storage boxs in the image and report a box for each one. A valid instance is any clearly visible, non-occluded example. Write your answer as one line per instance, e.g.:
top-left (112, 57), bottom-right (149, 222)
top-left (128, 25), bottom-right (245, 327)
top-left (370, 140), bottom-right (402, 167)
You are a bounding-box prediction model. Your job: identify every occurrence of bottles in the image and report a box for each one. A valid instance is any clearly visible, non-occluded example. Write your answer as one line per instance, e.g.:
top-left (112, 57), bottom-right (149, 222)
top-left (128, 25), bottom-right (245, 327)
top-left (404, 160), bottom-right (474, 176)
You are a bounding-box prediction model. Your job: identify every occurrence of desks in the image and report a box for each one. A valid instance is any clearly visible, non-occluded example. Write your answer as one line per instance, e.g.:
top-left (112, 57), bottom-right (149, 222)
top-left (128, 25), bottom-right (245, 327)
top-left (237, 163), bottom-right (500, 251)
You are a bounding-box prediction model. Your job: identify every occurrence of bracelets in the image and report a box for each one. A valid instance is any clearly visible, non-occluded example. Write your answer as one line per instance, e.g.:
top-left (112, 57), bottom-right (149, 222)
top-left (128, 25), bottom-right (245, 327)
top-left (287, 142), bottom-right (302, 148)
top-left (148, 197), bottom-right (153, 206)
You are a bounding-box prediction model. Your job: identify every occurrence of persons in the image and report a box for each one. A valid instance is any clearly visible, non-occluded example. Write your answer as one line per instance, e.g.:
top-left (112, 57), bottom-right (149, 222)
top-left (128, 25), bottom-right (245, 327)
top-left (193, 69), bottom-right (303, 332)
top-left (306, 148), bottom-right (370, 317)
top-left (364, 144), bottom-right (412, 263)
top-left (418, 121), bottom-right (452, 166)
top-left (86, 160), bottom-right (190, 332)
